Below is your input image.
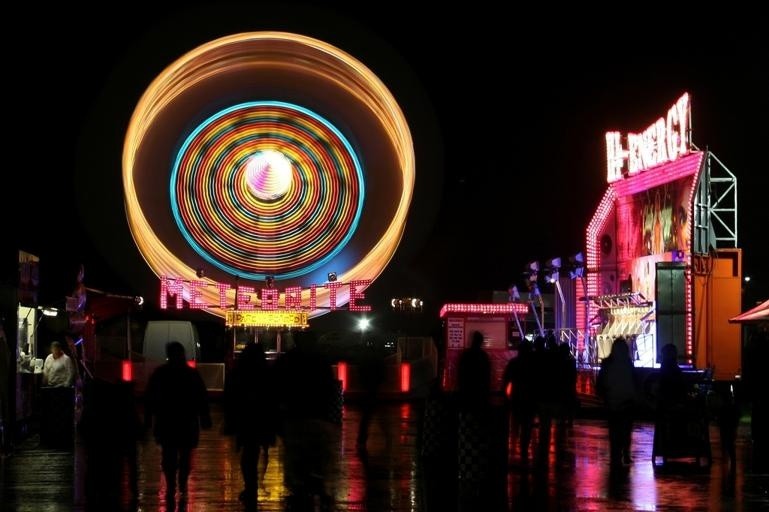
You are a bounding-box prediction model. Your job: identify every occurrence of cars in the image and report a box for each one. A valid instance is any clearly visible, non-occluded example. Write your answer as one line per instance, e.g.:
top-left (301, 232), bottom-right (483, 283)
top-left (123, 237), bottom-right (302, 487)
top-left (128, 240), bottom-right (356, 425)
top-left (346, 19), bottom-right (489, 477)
top-left (341, 342), bottom-right (371, 377)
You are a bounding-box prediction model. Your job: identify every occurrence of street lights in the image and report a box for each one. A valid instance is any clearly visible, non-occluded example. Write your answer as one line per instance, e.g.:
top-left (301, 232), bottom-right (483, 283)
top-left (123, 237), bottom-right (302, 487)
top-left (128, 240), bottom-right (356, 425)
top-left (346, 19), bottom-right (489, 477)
top-left (390, 297), bottom-right (424, 358)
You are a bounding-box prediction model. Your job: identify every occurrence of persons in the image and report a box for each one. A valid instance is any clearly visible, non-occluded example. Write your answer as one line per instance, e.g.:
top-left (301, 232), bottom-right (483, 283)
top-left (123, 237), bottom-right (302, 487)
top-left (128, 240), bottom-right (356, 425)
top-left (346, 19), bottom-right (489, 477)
top-left (42, 330), bottom-right (769, 512)
